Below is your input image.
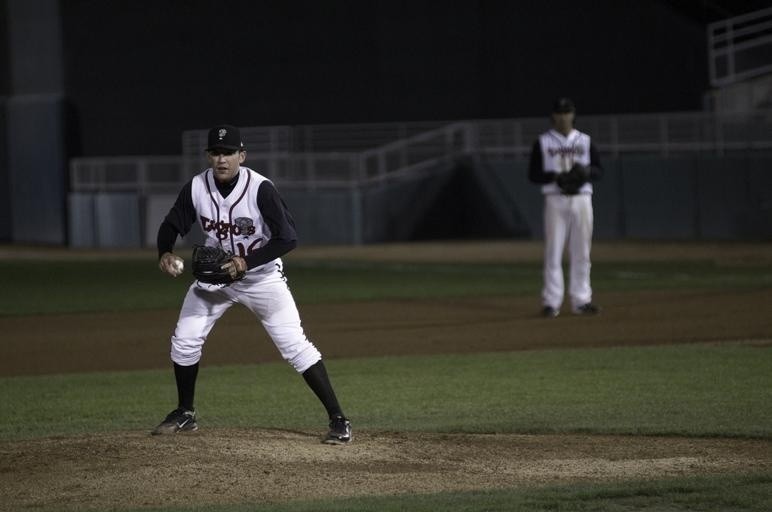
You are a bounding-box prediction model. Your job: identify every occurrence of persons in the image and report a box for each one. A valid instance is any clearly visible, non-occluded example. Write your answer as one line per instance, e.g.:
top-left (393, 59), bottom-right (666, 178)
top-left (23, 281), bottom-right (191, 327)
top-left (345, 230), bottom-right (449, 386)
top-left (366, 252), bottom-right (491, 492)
top-left (150, 123), bottom-right (353, 447)
top-left (524, 95), bottom-right (603, 317)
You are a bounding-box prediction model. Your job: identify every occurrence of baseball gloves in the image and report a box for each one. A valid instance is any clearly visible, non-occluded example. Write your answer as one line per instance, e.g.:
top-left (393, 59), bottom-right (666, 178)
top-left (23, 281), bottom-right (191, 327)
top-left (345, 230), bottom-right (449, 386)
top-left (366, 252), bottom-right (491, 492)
top-left (557, 162), bottom-right (588, 194)
top-left (190, 243), bottom-right (247, 288)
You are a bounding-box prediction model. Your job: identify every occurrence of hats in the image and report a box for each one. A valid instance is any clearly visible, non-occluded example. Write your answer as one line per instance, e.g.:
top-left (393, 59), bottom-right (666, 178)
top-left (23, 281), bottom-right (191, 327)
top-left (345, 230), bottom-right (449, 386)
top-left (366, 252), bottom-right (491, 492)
top-left (549, 96), bottom-right (576, 114)
top-left (204, 124), bottom-right (244, 151)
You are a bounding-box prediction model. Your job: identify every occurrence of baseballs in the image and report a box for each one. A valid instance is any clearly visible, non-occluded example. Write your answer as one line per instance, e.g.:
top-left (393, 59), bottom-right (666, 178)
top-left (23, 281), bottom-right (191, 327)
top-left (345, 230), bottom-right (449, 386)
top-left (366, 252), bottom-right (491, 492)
top-left (170, 259), bottom-right (184, 276)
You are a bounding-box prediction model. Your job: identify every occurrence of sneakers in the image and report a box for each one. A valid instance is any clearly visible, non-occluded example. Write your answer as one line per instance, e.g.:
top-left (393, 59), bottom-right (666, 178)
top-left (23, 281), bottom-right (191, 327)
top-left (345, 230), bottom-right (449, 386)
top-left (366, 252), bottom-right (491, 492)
top-left (152, 407), bottom-right (199, 435)
top-left (542, 306), bottom-right (561, 319)
top-left (573, 302), bottom-right (598, 316)
top-left (322, 416), bottom-right (353, 445)
